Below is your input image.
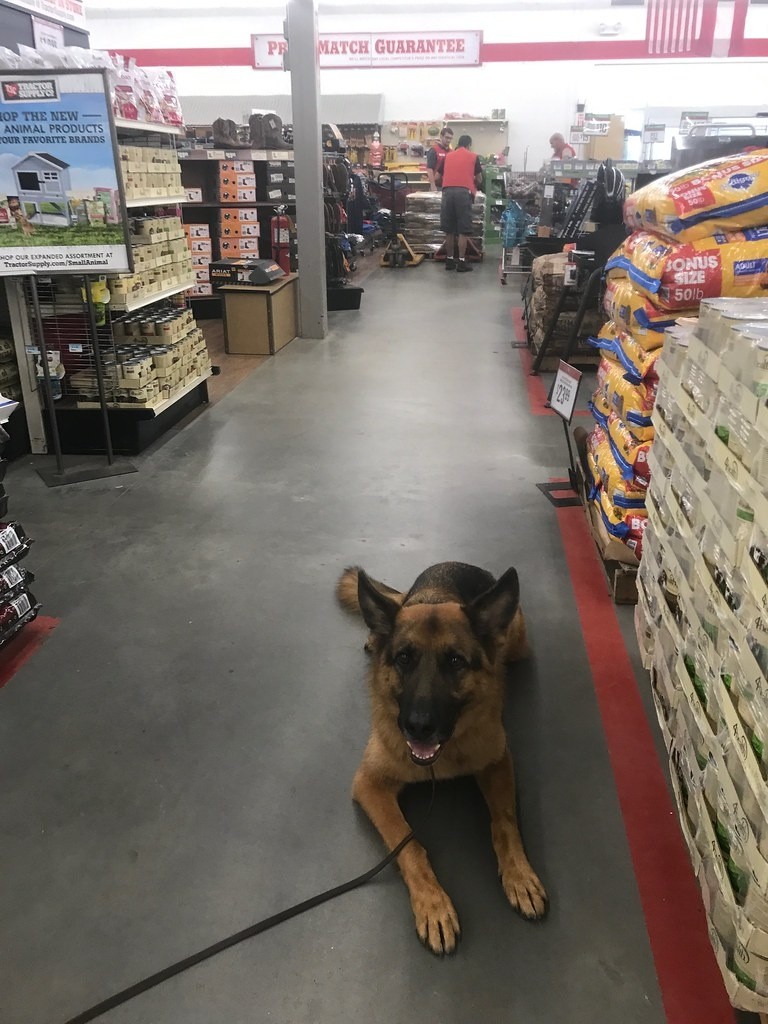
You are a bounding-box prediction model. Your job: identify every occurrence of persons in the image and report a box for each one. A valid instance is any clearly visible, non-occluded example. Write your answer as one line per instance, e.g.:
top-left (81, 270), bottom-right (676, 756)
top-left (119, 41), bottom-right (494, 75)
top-left (426, 128), bottom-right (455, 192)
top-left (435, 135), bottom-right (483, 272)
top-left (549, 133), bottom-right (578, 188)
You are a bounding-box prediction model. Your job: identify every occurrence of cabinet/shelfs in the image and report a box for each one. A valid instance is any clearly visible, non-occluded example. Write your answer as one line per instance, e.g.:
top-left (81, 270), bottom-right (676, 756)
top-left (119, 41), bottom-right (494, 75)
top-left (0, 118), bottom-right (213, 457)
top-left (369, 172), bottom-right (431, 212)
top-left (186, 148), bottom-right (296, 301)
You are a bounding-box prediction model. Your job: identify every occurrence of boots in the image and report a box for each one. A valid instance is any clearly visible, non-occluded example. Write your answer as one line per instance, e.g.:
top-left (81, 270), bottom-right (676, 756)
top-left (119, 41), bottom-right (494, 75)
top-left (213, 118), bottom-right (248, 148)
top-left (262, 114), bottom-right (294, 150)
top-left (249, 113), bottom-right (268, 150)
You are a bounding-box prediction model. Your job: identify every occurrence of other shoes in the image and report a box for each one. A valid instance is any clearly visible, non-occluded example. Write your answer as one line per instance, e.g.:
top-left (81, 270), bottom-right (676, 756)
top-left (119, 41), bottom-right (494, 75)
top-left (456, 259), bottom-right (473, 272)
top-left (446, 259), bottom-right (456, 270)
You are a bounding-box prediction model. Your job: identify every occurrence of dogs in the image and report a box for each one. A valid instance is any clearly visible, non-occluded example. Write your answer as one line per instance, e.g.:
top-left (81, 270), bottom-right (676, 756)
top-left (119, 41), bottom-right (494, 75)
top-left (336, 560), bottom-right (550, 960)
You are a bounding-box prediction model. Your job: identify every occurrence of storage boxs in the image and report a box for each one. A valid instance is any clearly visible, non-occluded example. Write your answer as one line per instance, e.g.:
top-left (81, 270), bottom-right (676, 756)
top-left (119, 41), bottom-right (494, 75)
top-left (328, 286), bottom-right (365, 311)
